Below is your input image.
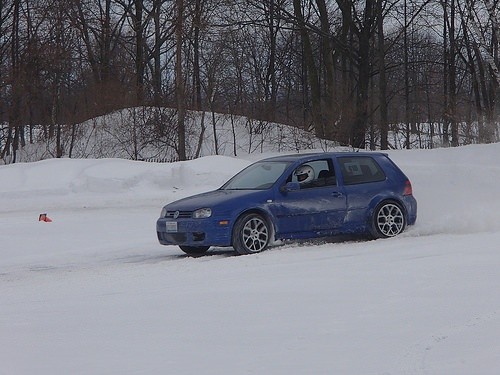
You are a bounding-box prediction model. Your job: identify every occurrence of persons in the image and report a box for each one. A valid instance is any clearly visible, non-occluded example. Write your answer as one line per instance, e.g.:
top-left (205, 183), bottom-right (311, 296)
top-left (294, 165), bottom-right (317, 189)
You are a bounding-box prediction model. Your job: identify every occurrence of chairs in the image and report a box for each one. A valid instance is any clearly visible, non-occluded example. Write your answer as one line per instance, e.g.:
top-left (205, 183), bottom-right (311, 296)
top-left (317, 170), bottom-right (334, 187)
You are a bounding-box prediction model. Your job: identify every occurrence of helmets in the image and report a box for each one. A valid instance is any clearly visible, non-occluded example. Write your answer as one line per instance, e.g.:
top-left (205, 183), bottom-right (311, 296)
top-left (294, 165), bottom-right (315, 184)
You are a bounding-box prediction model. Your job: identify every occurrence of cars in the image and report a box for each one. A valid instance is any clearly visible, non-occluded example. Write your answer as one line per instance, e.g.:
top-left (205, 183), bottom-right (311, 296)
top-left (156, 152), bottom-right (417, 256)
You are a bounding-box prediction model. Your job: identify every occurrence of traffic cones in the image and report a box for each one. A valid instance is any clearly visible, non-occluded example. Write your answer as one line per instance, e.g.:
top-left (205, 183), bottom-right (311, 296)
top-left (39, 213), bottom-right (52, 222)
